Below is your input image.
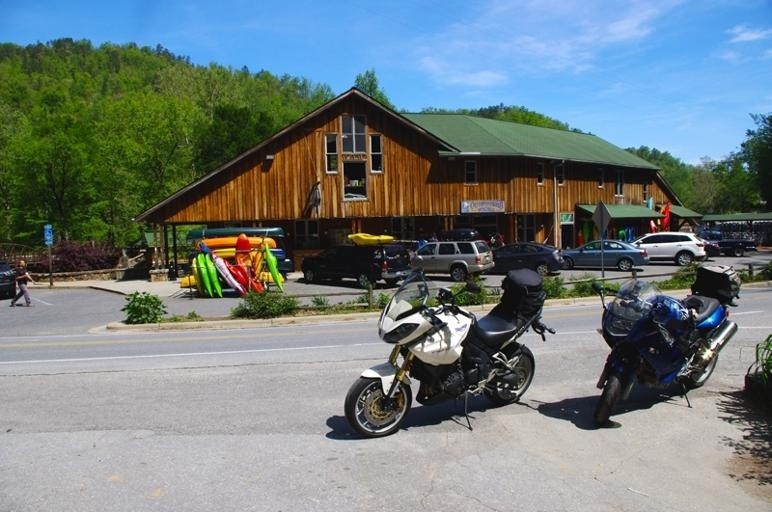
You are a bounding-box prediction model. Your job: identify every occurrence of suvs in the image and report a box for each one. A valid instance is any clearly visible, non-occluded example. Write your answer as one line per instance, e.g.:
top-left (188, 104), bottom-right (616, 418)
top-left (300, 242), bottom-right (413, 289)
top-left (411, 241), bottom-right (496, 282)
top-left (629, 232), bottom-right (706, 266)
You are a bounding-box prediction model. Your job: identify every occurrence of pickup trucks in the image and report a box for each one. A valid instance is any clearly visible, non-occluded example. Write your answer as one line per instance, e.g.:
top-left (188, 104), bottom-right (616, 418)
top-left (696, 230), bottom-right (756, 257)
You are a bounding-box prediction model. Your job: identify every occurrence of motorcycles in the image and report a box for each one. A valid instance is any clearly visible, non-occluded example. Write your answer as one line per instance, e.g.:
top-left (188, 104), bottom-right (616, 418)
top-left (343, 269), bottom-right (555, 438)
top-left (592, 266), bottom-right (742, 425)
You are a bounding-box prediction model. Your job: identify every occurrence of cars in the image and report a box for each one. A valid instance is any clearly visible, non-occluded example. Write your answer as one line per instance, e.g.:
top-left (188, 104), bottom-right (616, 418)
top-left (0, 261), bottom-right (16, 299)
top-left (562, 240), bottom-right (650, 272)
top-left (697, 237), bottom-right (720, 261)
top-left (487, 241), bottom-right (565, 276)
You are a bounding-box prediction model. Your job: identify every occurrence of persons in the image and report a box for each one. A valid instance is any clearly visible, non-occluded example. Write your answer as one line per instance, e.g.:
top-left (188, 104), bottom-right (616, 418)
top-left (12, 259), bottom-right (32, 306)
